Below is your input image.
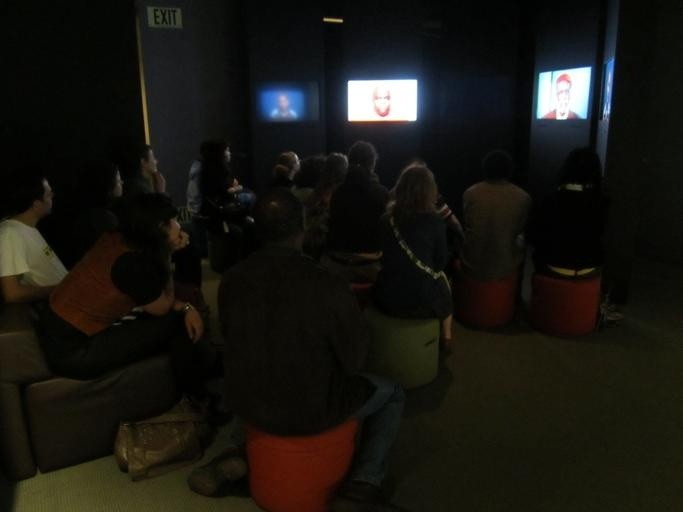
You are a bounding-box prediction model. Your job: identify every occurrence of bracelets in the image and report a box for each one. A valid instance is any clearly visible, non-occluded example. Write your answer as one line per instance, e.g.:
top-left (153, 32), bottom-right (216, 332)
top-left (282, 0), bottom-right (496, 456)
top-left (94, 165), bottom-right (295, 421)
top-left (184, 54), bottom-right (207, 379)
top-left (182, 302), bottom-right (194, 312)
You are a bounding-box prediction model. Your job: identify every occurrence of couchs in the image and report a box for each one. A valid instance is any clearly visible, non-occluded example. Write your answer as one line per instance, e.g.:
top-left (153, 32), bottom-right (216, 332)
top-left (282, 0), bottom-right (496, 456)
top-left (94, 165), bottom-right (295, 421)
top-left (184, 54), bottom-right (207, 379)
top-left (349, 279), bottom-right (375, 310)
top-left (365, 305), bottom-right (442, 389)
top-left (452, 260), bottom-right (524, 329)
top-left (1, 302), bottom-right (175, 485)
top-left (245, 417), bottom-right (355, 510)
top-left (528, 271), bottom-right (603, 342)
top-left (206, 228), bottom-right (251, 275)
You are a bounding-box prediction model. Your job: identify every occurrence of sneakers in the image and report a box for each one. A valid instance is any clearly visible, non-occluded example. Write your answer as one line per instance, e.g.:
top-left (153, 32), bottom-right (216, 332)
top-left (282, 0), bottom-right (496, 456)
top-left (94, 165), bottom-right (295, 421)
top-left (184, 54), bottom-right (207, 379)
top-left (439, 350), bottom-right (456, 370)
top-left (187, 454), bottom-right (247, 497)
top-left (341, 473), bottom-right (396, 503)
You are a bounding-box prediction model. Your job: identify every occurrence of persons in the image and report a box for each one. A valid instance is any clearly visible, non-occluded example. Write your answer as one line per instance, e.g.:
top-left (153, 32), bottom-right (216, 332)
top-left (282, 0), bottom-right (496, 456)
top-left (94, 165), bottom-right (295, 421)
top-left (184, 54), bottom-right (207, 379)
top-left (361, 86), bottom-right (399, 119)
top-left (184, 138), bottom-right (393, 277)
top-left (368, 162), bottom-right (469, 353)
top-left (186, 190), bottom-right (404, 504)
top-left (1, 137), bottom-right (211, 318)
top-left (529, 148), bottom-right (612, 281)
top-left (270, 94), bottom-right (298, 120)
top-left (37, 192), bottom-right (234, 430)
top-left (541, 73), bottom-right (581, 119)
top-left (463, 139), bottom-right (531, 310)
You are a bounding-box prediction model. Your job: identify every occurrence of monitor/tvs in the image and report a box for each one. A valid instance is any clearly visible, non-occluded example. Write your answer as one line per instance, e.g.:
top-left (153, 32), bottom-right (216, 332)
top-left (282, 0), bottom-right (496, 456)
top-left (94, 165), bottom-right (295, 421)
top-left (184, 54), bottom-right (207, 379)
top-left (257, 86), bottom-right (306, 122)
top-left (347, 79), bottom-right (418, 122)
top-left (536, 65), bottom-right (593, 120)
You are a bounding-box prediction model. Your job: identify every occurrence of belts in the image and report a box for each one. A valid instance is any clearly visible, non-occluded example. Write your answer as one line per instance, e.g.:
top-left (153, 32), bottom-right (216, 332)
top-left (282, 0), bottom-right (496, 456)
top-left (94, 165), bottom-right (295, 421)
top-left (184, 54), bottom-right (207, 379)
top-left (550, 266), bottom-right (595, 277)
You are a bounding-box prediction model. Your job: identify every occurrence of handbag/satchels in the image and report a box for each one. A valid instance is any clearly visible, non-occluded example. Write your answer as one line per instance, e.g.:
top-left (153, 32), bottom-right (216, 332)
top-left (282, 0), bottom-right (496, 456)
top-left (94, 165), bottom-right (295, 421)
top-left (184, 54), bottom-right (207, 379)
top-left (174, 282), bottom-right (210, 319)
top-left (595, 294), bottom-right (623, 342)
top-left (115, 398), bottom-right (205, 481)
top-left (326, 251), bottom-right (378, 282)
top-left (216, 187), bottom-right (257, 221)
